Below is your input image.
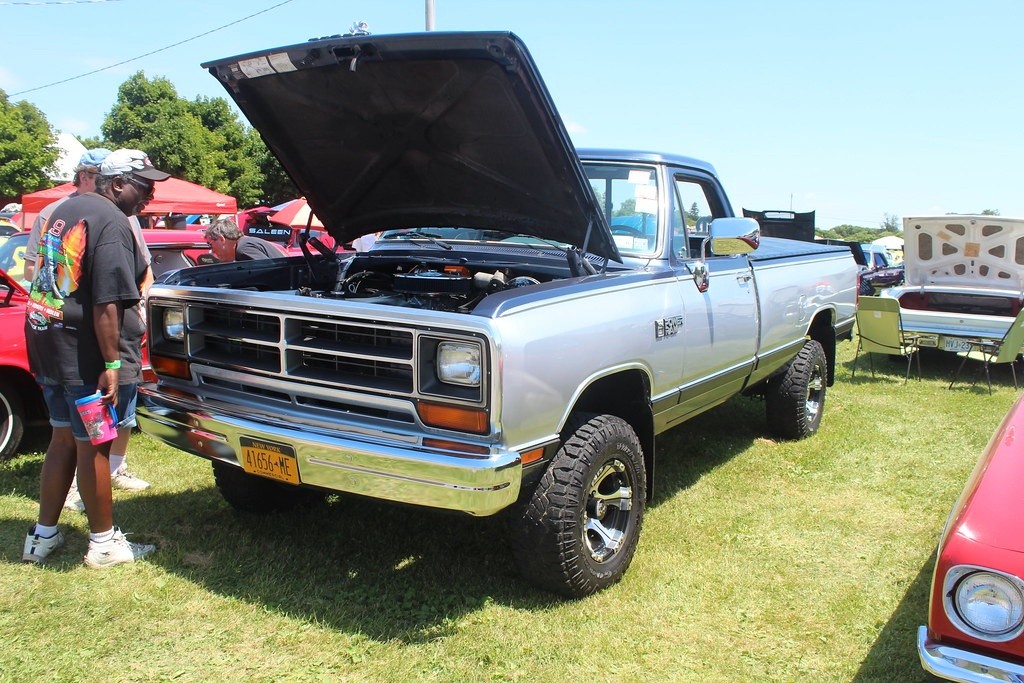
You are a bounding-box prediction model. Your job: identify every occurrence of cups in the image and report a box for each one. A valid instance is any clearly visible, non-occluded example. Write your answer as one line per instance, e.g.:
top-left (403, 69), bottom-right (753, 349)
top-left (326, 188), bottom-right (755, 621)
top-left (74, 392), bottom-right (118, 446)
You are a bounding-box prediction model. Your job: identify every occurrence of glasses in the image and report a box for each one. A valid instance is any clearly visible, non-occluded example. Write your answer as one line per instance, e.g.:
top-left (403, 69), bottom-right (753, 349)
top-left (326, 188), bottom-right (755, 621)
top-left (125, 176), bottom-right (155, 195)
top-left (207, 240), bottom-right (214, 246)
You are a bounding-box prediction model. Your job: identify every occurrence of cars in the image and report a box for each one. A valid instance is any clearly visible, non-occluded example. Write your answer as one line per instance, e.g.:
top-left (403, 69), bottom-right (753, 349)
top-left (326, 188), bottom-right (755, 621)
top-left (133, 27), bottom-right (861, 601)
top-left (1, 196), bottom-right (338, 461)
top-left (858, 243), bottom-right (892, 271)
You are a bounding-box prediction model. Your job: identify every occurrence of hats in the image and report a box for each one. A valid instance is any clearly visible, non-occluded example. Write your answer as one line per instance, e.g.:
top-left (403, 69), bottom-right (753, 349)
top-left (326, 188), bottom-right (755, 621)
top-left (77, 148), bottom-right (113, 168)
top-left (97, 148), bottom-right (171, 182)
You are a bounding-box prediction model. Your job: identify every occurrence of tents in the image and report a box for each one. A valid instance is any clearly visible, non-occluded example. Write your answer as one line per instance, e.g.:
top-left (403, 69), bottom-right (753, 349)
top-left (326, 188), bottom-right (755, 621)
top-left (22, 175), bottom-right (241, 230)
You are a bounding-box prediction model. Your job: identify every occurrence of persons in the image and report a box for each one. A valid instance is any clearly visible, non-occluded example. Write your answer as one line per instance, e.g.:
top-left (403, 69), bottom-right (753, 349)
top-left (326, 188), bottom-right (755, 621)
top-left (205, 219), bottom-right (284, 262)
top-left (24, 147), bottom-right (171, 569)
top-left (24, 147), bottom-right (157, 514)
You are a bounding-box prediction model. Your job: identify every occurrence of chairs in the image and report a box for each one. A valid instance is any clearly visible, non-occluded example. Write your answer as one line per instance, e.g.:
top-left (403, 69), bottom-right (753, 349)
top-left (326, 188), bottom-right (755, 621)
top-left (949, 307), bottom-right (1024, 396)
top-left (850, 295), bottom-right (920, 385)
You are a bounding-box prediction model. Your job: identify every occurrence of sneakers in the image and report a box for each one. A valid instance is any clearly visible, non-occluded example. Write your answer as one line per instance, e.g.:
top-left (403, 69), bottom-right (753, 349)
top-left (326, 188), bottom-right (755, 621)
top-left (63, 485), bottom-right (86, 512)
top-left (22, 521), bottom-right (65, 563)
top-left (84, 526), bottom-right (156, 569)
top-left (111, 463), bottom-right (151, 492)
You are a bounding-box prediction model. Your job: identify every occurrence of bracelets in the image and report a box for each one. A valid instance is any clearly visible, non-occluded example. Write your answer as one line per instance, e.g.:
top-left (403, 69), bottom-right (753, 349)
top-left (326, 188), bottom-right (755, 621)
top-left (105, 359), bottom-right (123, 369)
top-left (139, 297), bottom-right (147, 305)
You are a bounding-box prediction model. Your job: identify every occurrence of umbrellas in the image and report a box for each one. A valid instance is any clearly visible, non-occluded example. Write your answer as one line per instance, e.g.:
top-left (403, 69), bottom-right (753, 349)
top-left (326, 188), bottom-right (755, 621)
top-left (265, 197), bottom-right (325, 230)
